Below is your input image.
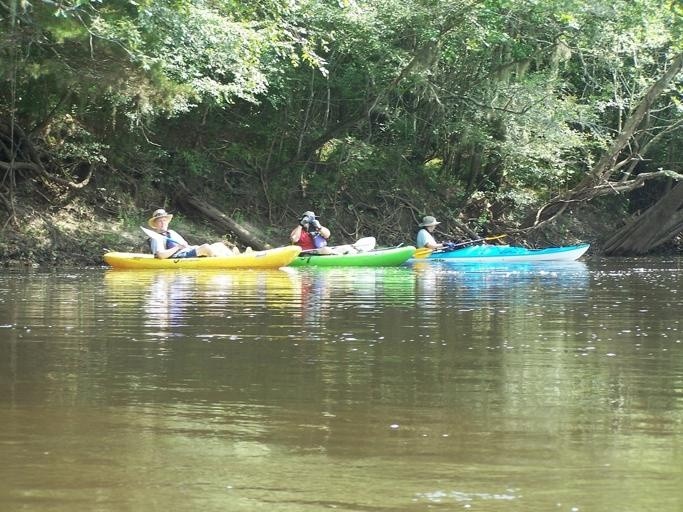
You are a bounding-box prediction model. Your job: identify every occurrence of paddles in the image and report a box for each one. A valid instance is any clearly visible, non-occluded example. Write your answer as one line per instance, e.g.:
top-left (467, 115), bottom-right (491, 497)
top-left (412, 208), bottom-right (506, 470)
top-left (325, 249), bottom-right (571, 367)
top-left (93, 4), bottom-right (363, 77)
top-left (413, 235), bottom-right (507, 258)
top-left (140, 225), bottom-right (187, 249)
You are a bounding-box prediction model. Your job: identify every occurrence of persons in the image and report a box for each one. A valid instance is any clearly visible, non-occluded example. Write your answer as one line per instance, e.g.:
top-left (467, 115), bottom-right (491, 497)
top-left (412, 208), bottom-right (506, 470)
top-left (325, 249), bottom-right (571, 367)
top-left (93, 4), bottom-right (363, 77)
top-left (290, 210), bottom-right (331, 252)
top-left (148, 208), bottom-right (253, 259)
top-left (416, 215), bottom-right (454, 249)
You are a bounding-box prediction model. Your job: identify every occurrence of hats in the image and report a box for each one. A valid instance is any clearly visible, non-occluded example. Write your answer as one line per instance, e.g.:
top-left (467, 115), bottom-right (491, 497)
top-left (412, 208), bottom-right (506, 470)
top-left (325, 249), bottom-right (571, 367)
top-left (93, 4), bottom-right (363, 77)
top-left (302, 211), bottom-right (314, 219)
top-left (417, 216), bottom-right (442, 226)
top-left (148, 208), bottom-right (173, 229)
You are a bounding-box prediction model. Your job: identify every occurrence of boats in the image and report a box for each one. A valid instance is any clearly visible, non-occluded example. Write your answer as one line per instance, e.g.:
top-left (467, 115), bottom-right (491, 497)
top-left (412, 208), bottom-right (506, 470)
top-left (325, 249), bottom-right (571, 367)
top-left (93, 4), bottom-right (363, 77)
top-left (102, 244), bottom-right (302, 272)
top-left (415, 243), bottom-right (591, 263)
top-left (287, 244), bottom-right (418, 268)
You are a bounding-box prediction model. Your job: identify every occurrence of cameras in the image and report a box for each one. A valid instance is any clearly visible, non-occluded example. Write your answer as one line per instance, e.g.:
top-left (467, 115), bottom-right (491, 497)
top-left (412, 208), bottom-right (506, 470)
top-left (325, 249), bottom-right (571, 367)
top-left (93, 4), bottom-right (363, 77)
top-left (309, 219), bottom-right (313, 224)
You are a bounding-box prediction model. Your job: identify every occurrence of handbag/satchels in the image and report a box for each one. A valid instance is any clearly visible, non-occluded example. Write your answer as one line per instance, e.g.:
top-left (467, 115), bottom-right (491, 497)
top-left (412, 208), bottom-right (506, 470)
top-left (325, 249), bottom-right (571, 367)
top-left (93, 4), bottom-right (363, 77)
top-left (313, 234), bottom-right (328, 248)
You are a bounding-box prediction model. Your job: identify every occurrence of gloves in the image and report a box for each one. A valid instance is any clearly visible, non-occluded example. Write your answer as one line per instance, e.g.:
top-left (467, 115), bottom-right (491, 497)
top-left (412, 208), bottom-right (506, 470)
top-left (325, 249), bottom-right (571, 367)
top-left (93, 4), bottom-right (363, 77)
top-left (312, 220), bottom-right (319, 227)
top-left (442, 242), bottom-right (456, 250)
top-left (300, 217), bottom-right (310, 226)
